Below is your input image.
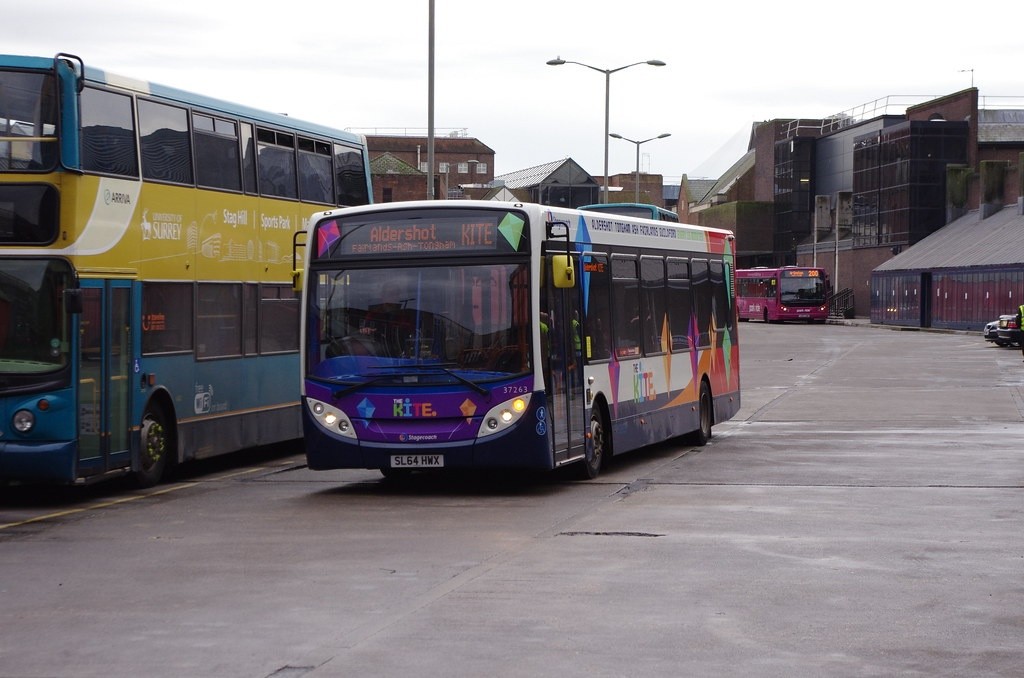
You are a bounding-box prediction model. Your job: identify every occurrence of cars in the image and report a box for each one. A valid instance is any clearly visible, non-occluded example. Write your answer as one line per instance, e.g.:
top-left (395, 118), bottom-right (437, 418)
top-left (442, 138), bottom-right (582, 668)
top-left (984, 320), bottom-right (1007, 348)
top-left (998, 312), bottom-right (1022, 347)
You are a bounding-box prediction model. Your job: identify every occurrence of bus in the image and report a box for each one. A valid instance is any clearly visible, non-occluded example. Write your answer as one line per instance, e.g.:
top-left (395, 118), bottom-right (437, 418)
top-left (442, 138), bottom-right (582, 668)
top-left (0, 50), bottom-right (376, 491)
top-left (578, 206), bottom-right (683, 224)
top-left (734, 266), bottom-right (834, 323)
top-left (290, 199), bottom-right (739, 482)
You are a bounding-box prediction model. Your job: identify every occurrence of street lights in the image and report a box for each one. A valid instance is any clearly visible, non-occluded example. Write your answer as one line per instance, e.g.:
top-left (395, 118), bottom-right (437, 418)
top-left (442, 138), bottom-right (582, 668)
top-left (444, 160), bottom-right (479, 198)
top-left (610, 132), bottom-right (673, 202)
top-left (547, 58), bottom-right (667, 202)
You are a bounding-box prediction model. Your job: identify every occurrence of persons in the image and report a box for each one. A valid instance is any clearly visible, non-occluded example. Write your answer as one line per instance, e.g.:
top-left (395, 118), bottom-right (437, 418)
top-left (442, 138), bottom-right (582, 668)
top-left (572, 310), bottom-right (581, 357)
top-left (357, 282), bottom-right (401, 335)
top-left (1016, 305), bottom-right (1024, 362)
top-left (540, 311), bottom-right (550, 357)
